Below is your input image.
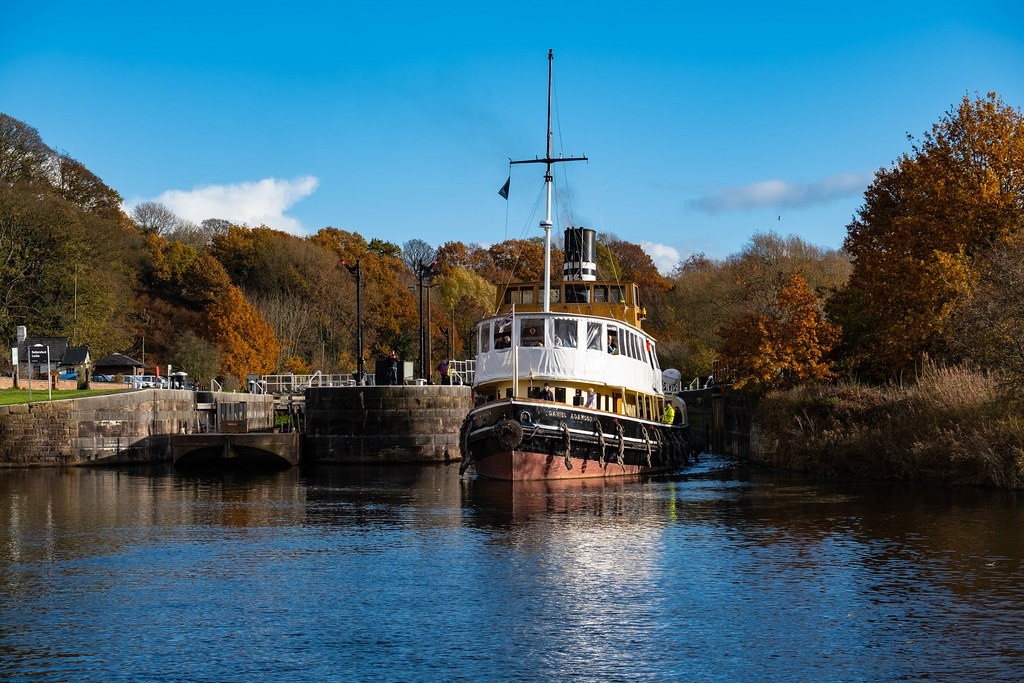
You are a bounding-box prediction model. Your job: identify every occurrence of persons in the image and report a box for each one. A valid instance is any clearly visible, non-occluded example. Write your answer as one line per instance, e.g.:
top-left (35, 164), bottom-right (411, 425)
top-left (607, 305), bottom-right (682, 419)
top-left (492, 329), bottom-right (618, 354)
top-left (385, 350), bottom-right (401, 386)
top-left (539, 383), bottom-right (554, 402)
top-left (660, 401), bottom-right (675, 425)
top-left (437, 357), bottom-right (451, 385)
top-left (584, 388), bottom-right (597, 409)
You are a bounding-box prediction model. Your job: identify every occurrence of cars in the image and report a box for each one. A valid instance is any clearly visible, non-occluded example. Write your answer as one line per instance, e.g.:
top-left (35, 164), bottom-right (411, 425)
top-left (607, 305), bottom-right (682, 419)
top-left (91, 374), bottom-right (185, 390)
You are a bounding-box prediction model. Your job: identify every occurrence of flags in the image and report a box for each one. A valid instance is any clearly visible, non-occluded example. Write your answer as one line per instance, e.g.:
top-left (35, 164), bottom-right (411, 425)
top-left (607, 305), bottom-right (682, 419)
top-left (498, 176), bottom-right (510, 200)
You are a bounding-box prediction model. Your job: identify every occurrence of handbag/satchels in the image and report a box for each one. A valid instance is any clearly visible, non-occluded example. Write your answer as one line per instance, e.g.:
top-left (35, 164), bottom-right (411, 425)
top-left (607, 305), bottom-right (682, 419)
top-left (437, 361), bottom-right (442, 372)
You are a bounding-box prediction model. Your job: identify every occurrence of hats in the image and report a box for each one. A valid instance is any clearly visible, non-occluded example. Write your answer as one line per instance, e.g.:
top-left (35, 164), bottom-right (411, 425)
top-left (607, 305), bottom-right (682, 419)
top-left (666, 401), bottom-right (670, 404)
top-left (544, 382), bottom-right (549, 386)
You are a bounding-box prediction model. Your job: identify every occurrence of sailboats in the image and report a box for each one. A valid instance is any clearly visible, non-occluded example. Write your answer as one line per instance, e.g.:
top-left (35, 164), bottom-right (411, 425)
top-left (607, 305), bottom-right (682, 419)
top-left (457, 50), bottom-right (692, 483)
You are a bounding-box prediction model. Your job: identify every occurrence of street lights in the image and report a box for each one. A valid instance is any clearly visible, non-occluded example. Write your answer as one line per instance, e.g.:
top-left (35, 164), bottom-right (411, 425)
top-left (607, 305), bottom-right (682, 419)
top-left (417, 257), bottom-right (438, 379)
top-left (469, 328), bottom-right (476, 360)
top-left (341, 258), bottom-right (364, 387)
top-left (436, 324), bottom-right (449, 366)
